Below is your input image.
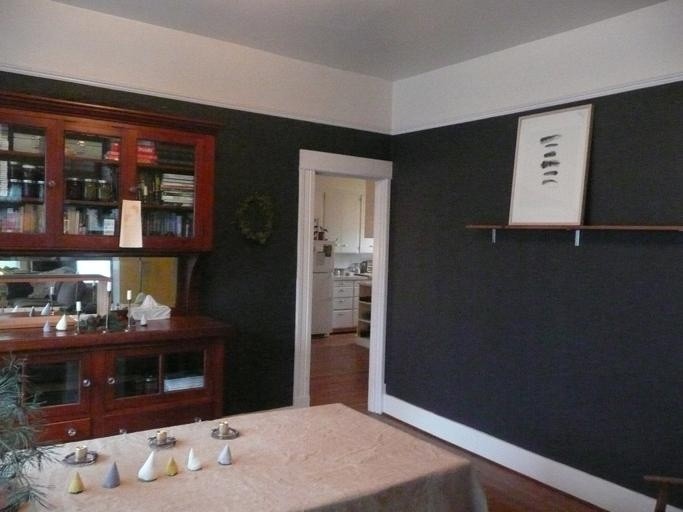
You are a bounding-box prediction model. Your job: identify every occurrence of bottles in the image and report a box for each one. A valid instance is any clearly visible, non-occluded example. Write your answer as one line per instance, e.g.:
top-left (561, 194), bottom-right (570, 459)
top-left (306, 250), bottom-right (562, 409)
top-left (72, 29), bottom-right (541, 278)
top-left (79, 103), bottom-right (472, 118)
top-left (8, 161), bottom-right (111, 200)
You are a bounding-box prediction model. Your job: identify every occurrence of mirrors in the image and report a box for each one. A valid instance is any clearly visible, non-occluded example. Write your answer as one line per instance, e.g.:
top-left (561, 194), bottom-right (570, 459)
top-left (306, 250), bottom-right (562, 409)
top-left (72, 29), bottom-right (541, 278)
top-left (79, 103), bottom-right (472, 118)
top-left (0, 272), bottom-right (110, 329)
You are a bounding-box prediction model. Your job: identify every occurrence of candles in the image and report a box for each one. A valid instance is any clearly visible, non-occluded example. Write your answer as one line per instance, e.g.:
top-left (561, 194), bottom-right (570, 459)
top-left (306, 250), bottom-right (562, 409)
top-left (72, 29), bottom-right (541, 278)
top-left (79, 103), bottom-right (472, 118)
top-left (126, 287), bottom-right (132, 301)
top-left (48, 286), bottom-right (54, 296)
top-left (74, 298), bottom-right (82, 311)
top-left (105, 280), bottom-right (112, 293)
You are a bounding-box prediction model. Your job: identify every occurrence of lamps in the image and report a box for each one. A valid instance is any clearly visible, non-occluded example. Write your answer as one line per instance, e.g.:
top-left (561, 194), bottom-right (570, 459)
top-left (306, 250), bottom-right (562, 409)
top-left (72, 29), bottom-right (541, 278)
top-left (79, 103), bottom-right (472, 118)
top-left (117, 182), bottom-right (145, 250)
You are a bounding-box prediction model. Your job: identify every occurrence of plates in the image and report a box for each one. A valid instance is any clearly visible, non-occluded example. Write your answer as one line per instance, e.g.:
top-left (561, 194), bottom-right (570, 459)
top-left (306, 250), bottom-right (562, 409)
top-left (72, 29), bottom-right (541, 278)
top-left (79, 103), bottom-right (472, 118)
top-left (211, 426), bottom-right (239, 439)
top-left (149, 436), bottom-right (175, 447)
top-left (61, 452), bottom-right (98, 468)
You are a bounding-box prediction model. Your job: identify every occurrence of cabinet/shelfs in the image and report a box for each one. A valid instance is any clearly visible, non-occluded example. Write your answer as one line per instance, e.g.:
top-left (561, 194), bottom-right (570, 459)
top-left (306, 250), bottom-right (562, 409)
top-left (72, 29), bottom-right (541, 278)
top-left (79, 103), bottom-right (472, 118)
top-left (333, 278), bottom-right (368, 329)
top-left (315, 174), bottom-right (366, 254)
top-left (0, 106), bottom-right (218, 254)
top-left (355, 280), bottom-right (371, 349)
top-left (0, 312), bottom-right (233, 450)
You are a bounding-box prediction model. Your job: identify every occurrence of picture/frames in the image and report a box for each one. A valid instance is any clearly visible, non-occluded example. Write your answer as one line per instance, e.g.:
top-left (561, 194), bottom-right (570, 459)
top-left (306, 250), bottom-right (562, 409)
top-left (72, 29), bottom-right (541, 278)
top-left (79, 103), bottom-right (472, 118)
top-left (506, 101), bottom-right (598, 228)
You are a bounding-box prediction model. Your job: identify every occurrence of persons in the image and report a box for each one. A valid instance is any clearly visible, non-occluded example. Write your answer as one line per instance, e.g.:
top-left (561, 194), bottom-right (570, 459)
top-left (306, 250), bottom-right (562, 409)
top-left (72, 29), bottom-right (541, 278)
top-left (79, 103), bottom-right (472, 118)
top-left (49, 260), bottom-right (95, 314)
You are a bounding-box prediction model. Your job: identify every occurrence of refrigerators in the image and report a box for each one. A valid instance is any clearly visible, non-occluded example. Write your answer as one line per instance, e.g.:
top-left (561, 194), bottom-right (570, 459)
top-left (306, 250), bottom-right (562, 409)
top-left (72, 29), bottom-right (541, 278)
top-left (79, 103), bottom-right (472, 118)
top-left (310, 239), bottom-right (335, 338)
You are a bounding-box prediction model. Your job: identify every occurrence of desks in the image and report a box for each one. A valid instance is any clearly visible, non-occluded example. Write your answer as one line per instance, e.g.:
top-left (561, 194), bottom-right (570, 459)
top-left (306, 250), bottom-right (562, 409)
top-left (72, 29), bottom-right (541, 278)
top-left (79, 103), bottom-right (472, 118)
top-left (0, 397), bottom-right (491, 512)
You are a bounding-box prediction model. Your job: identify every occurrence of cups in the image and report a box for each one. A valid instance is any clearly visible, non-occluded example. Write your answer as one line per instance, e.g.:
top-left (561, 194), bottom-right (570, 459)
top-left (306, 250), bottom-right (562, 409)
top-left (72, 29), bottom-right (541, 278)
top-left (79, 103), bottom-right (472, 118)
top-left (154, 430), bottom-right (166, 441)
top-left (218, 421), bottom-right (230, 435)
top-left (74, 444), bottom-right (86, 460)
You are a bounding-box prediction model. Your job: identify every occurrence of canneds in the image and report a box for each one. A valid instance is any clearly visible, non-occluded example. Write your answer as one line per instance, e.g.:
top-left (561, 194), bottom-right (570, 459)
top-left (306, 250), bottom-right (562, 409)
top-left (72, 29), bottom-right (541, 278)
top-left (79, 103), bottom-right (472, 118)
top-left (8, 161), bottom-right (112, 202)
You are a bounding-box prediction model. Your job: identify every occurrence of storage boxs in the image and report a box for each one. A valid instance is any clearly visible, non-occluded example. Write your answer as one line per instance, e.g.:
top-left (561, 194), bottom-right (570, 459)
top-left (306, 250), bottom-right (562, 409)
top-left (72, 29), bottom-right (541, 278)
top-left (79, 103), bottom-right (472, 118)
top-left (11, 132), bottom-right (46, 156)
top-left (63, 138), bottom-right (104, 162)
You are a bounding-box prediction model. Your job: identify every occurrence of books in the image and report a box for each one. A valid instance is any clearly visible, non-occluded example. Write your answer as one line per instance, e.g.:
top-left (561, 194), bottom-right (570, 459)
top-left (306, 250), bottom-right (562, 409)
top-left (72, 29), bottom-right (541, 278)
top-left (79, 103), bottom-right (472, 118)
top-left (0, 123), bottom-right (195, 238)
